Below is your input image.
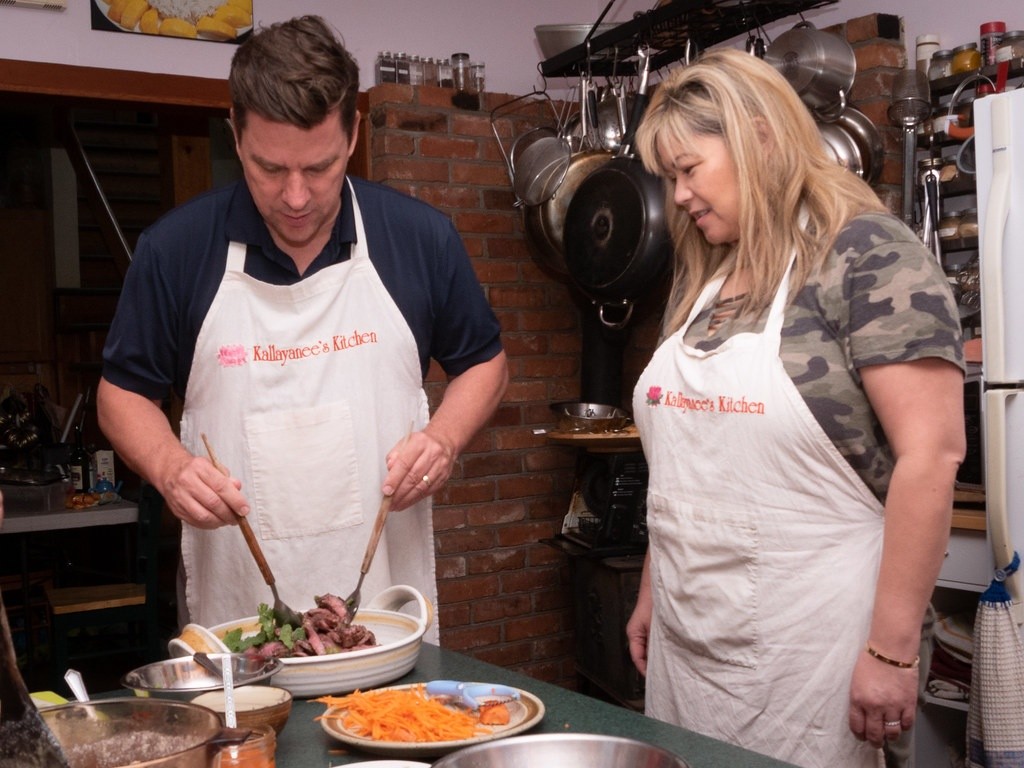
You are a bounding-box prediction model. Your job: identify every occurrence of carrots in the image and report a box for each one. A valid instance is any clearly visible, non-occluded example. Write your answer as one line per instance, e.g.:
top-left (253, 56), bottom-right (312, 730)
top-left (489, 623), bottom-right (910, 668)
top-left (308, 680), bottom-right (509, 755)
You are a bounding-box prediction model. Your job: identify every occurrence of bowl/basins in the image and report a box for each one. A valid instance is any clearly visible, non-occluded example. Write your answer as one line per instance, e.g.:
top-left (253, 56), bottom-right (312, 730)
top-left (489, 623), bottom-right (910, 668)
top-left (37, 697), bottom-right (223, 768)
top-left (190, 684), bottom-right (292, 736)
top-left (534, 21), bottom-right (624, 59)
top-left (429, 732), bottom-right (691, 768)
top-left (119, 652), bottom-right (284, 704)
top-left (166, 584), bottom-right (433, 697)
top-left (548, 403), bottom-right (629, 431)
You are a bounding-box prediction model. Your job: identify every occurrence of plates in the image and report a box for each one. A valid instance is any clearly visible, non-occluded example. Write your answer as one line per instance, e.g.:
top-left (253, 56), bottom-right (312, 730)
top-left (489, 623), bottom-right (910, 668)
top-left (320, 681), bottom-right (545, 758)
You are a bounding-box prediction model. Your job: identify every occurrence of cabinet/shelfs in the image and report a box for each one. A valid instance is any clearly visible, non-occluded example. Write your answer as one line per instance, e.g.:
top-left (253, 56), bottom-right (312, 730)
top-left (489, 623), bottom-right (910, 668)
top-left (912, 507), bottom-right (989, 768)
top-left (909, 56), bottom-right (1024, 384)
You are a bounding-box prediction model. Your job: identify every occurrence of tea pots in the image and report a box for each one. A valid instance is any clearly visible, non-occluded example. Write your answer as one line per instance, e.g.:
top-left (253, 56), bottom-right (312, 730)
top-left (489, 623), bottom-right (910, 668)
top-left (89, 477), bottom-right (124, 494)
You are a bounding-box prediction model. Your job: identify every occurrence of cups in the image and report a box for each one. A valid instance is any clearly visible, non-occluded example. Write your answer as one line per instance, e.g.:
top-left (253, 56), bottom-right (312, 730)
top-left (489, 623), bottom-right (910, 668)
top-left (215, 721), bottom-right (276, 768)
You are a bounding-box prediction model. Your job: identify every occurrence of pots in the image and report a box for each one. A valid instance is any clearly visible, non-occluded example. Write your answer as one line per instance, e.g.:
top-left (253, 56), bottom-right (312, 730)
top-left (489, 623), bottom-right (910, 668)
top-left (524, 22), bottom-right (883, 331)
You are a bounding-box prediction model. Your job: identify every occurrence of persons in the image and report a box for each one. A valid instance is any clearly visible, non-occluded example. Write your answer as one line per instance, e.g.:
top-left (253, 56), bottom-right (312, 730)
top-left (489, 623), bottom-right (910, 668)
top-left (626, 45), bottom-right (967, 768)
top-left (98, 13), bottom-right (508, 645)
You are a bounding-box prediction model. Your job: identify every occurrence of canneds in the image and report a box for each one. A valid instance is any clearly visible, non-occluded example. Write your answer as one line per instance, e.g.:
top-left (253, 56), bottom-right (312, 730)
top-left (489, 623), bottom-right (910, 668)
top-left (918, 83), bottom-right (997, 295)
top-left (927, 20), bottom-right (1024, 80)
top-left (376, 51), bottom-right (485, 92)
top-left (208, 721), bottom-right (277, 768)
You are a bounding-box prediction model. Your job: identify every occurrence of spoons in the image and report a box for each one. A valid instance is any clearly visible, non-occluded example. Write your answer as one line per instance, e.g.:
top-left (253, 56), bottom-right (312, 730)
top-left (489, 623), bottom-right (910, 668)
top-left (203, 433), bottom-right (301, 631)
top-left (344, 421), bottom-right (414, 622)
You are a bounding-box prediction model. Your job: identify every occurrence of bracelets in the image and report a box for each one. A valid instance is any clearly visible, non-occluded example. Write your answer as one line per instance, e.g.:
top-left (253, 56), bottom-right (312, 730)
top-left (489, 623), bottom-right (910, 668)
top-left (865, 641), bottom-right (920, 669)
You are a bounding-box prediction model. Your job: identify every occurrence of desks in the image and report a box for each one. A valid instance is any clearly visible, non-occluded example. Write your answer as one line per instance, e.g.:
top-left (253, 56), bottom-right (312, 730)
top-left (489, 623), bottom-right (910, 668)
top-left (0, 484), bottom-right (140, 700)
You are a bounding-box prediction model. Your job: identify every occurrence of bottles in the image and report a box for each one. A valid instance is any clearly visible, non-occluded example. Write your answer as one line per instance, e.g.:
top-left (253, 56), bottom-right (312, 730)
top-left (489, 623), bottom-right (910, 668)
top-left (374, 50), bottom-right (486, 92)
top-left (71, 424), bottom-right (96, 494)
top-left (915, 21), bottom-right (1024, 301)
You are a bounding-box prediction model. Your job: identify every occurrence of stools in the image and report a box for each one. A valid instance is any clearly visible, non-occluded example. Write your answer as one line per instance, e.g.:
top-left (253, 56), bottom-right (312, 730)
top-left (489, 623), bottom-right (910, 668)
top-left (43, 577), bottom-right (147, 691)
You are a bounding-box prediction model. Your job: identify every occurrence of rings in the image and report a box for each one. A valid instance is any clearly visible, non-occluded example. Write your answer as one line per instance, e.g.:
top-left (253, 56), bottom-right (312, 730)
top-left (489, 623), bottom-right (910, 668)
top-left (885, 720), bottom-right (901, 726)
top-left (423, 475), bottom-right (431, 487)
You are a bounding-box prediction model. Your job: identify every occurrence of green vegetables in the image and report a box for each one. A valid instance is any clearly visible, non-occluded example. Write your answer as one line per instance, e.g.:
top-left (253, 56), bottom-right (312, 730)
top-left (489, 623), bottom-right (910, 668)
top-left (226, 603), bottom-right (304, 652)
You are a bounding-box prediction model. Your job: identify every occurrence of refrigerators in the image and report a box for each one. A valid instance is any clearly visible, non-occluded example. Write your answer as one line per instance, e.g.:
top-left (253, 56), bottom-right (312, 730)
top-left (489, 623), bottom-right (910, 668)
top-left (973, 89), bottom-right (1024, 612)
